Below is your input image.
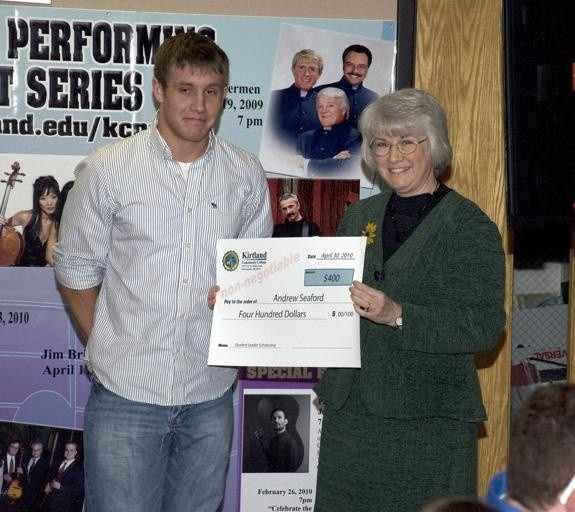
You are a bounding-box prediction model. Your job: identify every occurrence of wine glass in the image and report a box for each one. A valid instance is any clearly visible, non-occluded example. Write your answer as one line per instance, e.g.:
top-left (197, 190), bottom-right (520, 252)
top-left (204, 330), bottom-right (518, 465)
top-left (365, 303), bottom-right (373, 312)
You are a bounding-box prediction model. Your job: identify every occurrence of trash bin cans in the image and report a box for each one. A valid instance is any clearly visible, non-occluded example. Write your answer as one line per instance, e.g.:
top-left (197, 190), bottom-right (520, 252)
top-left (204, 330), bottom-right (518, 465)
top-left (391, 313), bottom-right (404, 331)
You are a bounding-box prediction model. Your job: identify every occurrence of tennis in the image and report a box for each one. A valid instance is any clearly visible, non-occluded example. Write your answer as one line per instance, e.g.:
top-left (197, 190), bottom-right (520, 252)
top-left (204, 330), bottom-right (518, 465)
top-left (0, 161), bottom-right (27, 267)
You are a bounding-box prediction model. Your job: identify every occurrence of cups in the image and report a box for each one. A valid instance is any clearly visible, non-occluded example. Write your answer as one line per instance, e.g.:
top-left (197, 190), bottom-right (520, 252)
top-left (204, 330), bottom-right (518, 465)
top-left (369, 138), bottom-right (429, 157)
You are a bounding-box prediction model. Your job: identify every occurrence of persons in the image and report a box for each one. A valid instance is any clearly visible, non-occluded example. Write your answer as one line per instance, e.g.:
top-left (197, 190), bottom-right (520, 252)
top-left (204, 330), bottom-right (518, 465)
top-left (289, 87), bottom-right (364, 180)
top-left (267, 50), bottom-right (325, 152)
top-left (0, 438), bottom-right (84, 510)
top-left (480, 378), bottom-right (575, 511)
top-left (311, 42), bottom-right (384, 129)
top-left (256, 409), bottom-right (305, 474)
top-left (43, 181), bottom-right (75, 267)
top-left (270, 192), bottom-right (324, 238)
top-left (49, 30), bottom-right (278, 510)
top-left (0, 175), bottom-right (60, 267)
top-left (205, 85), bottom-right (511, 511)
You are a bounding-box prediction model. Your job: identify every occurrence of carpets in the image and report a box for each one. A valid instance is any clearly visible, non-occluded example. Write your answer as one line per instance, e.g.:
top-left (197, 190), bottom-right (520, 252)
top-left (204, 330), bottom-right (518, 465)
top-left (60, 463), bottom-right (66, 471)
top-left (10, 458), bottom-right (13, 474)
